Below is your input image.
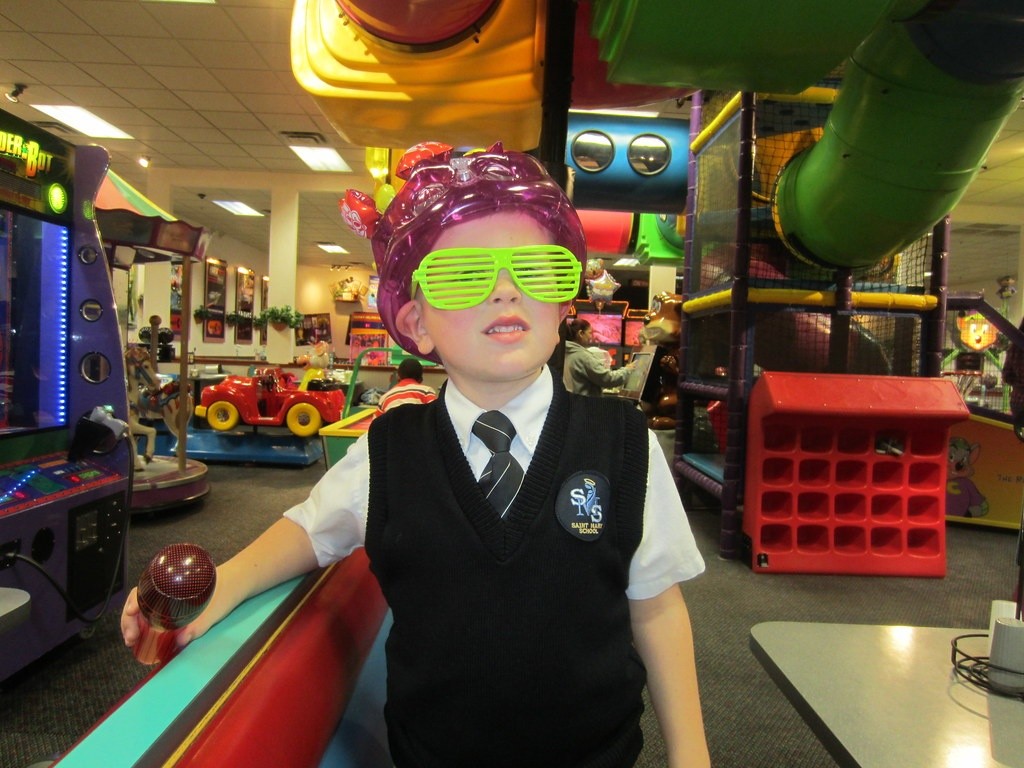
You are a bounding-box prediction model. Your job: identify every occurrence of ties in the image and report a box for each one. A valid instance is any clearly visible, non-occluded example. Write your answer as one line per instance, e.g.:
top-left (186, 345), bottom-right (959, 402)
top-left (470, 410), bottom-right (524, 519)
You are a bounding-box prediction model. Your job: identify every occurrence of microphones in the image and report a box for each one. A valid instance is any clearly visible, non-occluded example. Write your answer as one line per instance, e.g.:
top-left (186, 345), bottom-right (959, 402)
top-left (134, 543), bottom-right (215, 664)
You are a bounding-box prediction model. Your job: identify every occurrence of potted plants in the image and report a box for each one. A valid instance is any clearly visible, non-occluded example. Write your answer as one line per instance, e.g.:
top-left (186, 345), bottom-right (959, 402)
top-left (194, 305), bottom-right (303, 332)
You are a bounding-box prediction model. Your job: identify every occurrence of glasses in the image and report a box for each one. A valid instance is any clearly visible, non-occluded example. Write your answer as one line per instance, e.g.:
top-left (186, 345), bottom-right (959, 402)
top-left (409, 245), bottom-right (583, 310)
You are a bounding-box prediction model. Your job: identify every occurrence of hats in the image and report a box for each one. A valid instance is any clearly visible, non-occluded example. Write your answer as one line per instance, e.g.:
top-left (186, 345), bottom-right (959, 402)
top-left (342, 141), bottom-right (587, 360)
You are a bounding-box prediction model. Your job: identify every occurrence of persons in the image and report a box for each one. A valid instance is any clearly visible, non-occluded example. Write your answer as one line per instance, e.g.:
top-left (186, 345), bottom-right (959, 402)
top-left (372, 358), bottom-right (437, 420)
top-left (119, 141), bottom-right (714, 768)
top-left (562, 317), bottom-right (639, 396)
top-left (1002, 319), bottom-right (1024, 426)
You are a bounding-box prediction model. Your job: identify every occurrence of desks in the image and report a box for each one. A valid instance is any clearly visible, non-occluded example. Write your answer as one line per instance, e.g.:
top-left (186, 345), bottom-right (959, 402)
top-left (749, 621), bottom-right (1024, 768)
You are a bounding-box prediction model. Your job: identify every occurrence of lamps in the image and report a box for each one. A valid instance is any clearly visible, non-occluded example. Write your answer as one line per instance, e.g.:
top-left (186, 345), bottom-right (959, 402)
top-left (6, 84), bottom-right (27, 102)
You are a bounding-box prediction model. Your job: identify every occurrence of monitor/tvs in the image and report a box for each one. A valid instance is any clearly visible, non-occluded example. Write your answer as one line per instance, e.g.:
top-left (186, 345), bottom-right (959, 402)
top-left (618, 352), bottom-right (655, 399)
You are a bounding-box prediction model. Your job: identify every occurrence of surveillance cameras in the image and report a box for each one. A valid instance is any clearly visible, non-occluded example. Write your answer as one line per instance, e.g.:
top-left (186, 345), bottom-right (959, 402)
top-left (198, 194), bottom-right (208, 199)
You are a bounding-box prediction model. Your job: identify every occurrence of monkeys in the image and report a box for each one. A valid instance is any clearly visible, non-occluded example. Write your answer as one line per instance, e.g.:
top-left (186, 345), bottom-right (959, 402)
top-left (641, 289), bottom-right (685, 431)
top-left (956, 310), bottom-right (999, 350)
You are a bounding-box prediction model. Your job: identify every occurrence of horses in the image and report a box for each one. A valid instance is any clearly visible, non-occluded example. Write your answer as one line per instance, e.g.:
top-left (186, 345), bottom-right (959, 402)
top-left (125, 344), bottom-right (194, 472)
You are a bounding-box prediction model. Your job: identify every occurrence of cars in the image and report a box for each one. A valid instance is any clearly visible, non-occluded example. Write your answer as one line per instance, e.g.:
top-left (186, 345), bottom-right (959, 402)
top-left (193, 365), bottom-right (347, 439)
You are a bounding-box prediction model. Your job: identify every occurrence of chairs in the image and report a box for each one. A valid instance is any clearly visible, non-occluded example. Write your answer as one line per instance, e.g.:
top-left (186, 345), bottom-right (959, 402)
top-left (295, 368), bottom-right (326, 391)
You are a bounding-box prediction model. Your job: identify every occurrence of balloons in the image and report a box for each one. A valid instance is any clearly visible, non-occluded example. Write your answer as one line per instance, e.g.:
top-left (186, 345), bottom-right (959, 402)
top-left (583, 258), bottom-right (620, 303)
